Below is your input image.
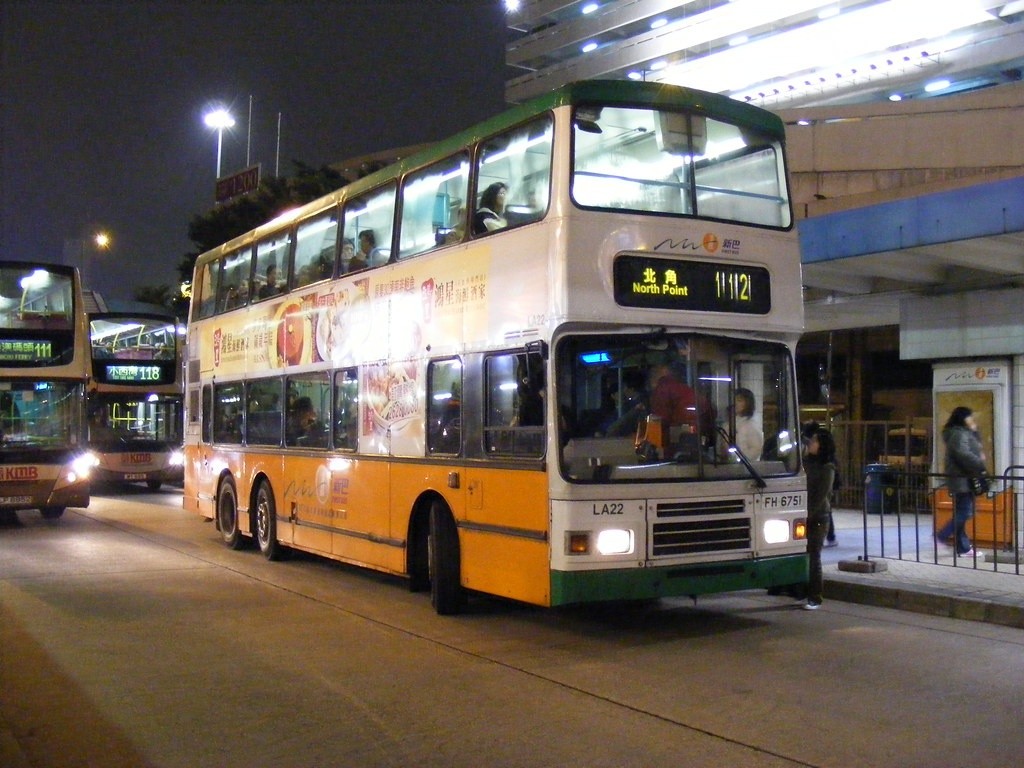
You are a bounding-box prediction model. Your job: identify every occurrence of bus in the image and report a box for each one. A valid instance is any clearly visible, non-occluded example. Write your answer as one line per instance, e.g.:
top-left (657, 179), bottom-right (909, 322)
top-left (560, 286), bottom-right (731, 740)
top-left (90, 311), bottom-right (182, 493)
top-left (0, 256), bottom-right (90, 521)
top-left (184, 81), bottom-right (815, 626)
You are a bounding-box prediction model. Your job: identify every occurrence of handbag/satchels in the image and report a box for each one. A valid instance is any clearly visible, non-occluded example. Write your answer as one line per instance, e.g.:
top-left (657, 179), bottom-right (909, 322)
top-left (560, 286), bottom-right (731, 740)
top-left (967, 469), bottom-right (993, 496)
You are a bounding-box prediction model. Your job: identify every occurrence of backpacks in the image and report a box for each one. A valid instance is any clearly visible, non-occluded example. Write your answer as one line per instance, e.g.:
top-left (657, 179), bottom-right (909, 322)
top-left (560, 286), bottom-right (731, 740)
top-left (832, 465), bottom-right (842, 490)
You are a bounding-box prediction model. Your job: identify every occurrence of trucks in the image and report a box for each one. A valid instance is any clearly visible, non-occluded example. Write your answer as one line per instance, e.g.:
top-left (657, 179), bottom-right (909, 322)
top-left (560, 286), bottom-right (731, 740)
top-left (878, 412), bottom-right (932, 497)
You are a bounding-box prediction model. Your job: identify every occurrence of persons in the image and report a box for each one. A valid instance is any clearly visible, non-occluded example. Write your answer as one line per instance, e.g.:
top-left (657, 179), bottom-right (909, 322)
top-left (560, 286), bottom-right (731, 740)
top-left (227, 230), bottom-right (389, 308)
top-left (435, 359), bottom-right (544, 453)
top-left (473, 181), bottom-right (508, 234)
top-left (153, 343), bottom-right (173, 360)
top-left (716, 388), bottom-right (763, 462)
top-left (445, 208), bottom-right (467, 243)
top-left (594, 362), bottom-right (717, 466)
top-left (938, 407), bottom-right (990, 557)
top-left (95, 341), bottom-right (116, 358)
top-left (288, 397), bottom-right (317, 441)
top-left (760, 422), bottom-right (840, 610)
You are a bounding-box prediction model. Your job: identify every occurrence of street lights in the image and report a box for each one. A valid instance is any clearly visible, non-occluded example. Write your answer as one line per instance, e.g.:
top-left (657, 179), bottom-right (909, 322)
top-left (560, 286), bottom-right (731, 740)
top-left (205, 110), bottom-right (235, 205)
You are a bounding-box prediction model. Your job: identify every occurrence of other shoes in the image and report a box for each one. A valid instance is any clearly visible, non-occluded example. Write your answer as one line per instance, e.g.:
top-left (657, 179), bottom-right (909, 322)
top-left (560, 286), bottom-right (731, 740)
top-left (958, 548), bottom-right (983, 558)
top-left (804, 601), bottom-right (822, 610)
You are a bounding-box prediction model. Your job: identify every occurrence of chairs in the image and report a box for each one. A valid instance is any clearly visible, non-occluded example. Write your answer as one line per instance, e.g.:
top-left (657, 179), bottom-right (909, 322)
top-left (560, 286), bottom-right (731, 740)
top-left (11, 309), bottom-right (66, 329)
top-left (93, 342), bottom-right (156, 358)
top-left (504, 204), bottom-right (545, 225)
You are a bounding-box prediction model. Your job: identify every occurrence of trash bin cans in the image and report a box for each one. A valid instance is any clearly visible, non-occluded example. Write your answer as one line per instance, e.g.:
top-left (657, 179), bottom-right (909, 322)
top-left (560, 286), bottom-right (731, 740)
top-left (865, 464), bottom-right (896, 514)
